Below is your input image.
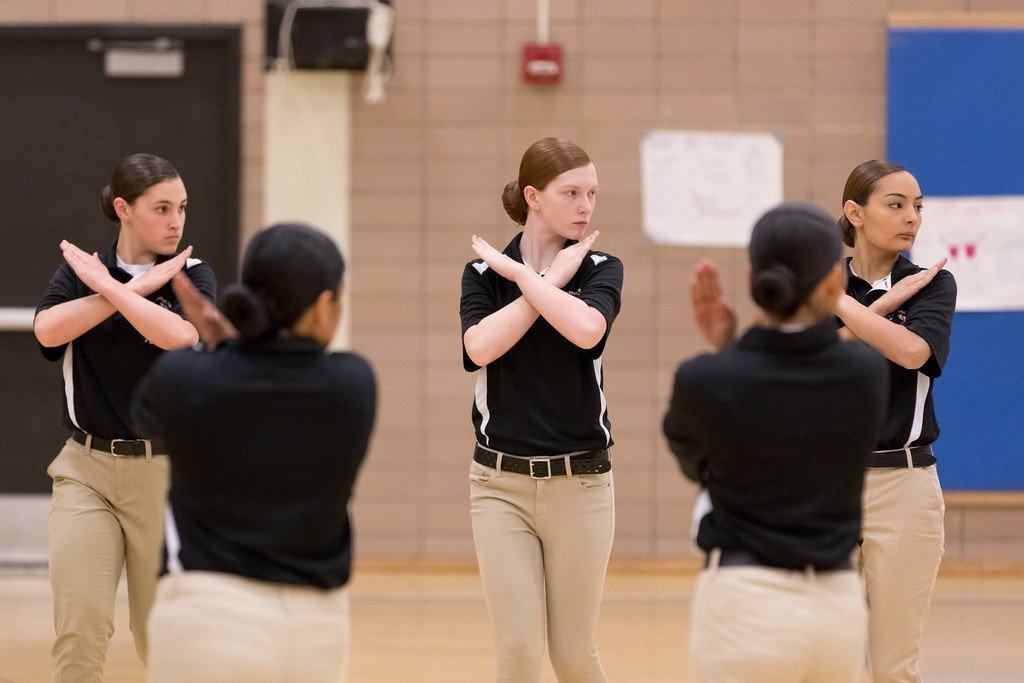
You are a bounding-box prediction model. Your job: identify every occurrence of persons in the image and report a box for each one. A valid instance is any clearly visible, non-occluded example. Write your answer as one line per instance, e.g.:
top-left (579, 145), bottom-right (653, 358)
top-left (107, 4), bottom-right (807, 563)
top-left (836, 157), bottom-right (959, 683)
top-left (460, 135), bottom-right (626, 683)
top-left (130, 220), bottom-right (378, 683)
top-left (658, 198), bottom-right (891, 683)
top-left (33, 152), bottom-right (218, 683)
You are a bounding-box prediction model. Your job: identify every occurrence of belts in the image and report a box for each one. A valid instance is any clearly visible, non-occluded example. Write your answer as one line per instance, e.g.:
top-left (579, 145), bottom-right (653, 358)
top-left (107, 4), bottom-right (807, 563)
top-left (705, 552), bottom-right (850, 570)
top-left (473, 441), bottom-right (612, 479)
top-left (71, 430), bottom-right (165, 457)
top-left (866, 444), bottom-right (937, 468)
